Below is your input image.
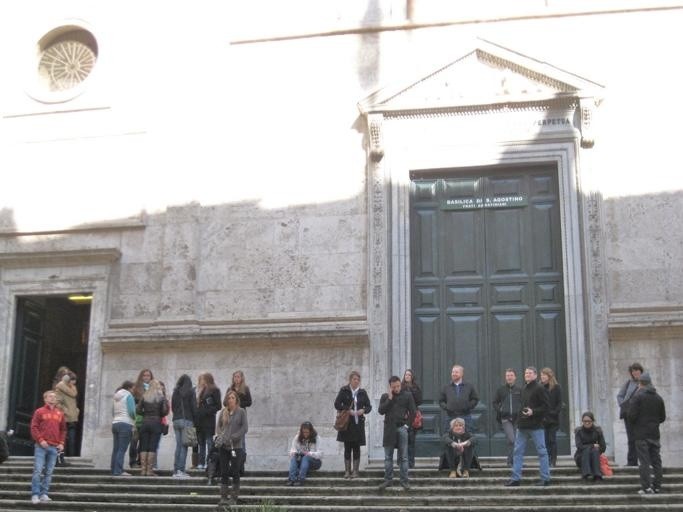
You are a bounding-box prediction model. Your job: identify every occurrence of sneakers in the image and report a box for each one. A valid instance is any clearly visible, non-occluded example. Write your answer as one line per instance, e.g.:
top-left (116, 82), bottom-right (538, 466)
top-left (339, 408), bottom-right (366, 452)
top-left (637, 487), bottom-right (654, 494)
top-left (40, 494), bottom-right (52, 502)
top-left (32, 494), bottom-right (40, 504)
top-left (449, 469), bottom-right (470, 478)
top-left (172, 470), bottom-right (190, 477)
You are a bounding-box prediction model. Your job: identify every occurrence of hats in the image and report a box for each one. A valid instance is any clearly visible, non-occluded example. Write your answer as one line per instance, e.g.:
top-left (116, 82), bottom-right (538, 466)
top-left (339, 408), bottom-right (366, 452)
top-left (639, 372), bottom-right (651, 381)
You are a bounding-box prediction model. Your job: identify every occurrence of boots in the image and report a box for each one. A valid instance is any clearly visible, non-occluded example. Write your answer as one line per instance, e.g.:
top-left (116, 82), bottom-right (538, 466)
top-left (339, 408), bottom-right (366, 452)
top-left (139, 451), bottom-right (147, 476)
top-left (226, 482), bottom-right (241, 504)
top-left (217, 482), bottom-right (229, 505)
top-left (350, 458), bottom-right (360, 478)
top-left (344, 458), bottom-right (351, 478)
top-left (146, 451), bottom-right (159, 477)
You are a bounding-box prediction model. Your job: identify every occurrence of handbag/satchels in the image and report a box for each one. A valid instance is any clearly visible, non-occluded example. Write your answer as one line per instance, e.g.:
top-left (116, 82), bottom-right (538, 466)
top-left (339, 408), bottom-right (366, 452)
top-left (599, 453), bottom-right (610, 476)
top-left (333, 409), bottom-right (350, 432)
top-left (619, 402), bottom-right (632, 419)
top-left (181, 426), bottom-right (198, 446)
top-left (406, 410), bottom-right (423, 429)
top-left (214, 435), bottom-right (224, 449)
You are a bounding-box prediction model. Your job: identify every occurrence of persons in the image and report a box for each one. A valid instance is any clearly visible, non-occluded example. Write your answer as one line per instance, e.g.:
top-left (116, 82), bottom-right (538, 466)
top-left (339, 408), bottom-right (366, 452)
top-left (30, 389), bottom-right (67, 504)
top-left (491, 367), bottom-right (526, 468)
top-left (437, 364), bottom-right (479, 433)
top-left (110, 368), bottom-right (253, 484)
top-left (214, 390), bottom-right (248, 505)
top-left (572, 411), bottom-right (607, 480)
top-left (284, 421), bottom-right (323, 487)
top-left (615, 362), bottom-right (645, 466)
top-left (395, 369), bottom-right (422, 468)
top-left (503, 365), bottom-right (551, 486)
top-left (377, 375), bottom-right (417, 490)
top-left (51, 365), bottom-right (68, 389)
top-left (56, 370), bottom-right (79, 456)
top-left (625, 370), bottom-right (666, 495)
top-left (437, 416), bottom-right (482, 478)
top-left (334, 371), bottom-right (372, 478)
top-left (536, 367), bottom-right (563, 468)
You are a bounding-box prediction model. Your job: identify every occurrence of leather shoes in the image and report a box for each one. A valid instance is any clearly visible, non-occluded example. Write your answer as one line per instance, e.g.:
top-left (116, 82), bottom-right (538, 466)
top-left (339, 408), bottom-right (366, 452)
top-left (503, 480), bottom-right (520, 486)
top-left (536, 480), bottom-right (548, 486)
